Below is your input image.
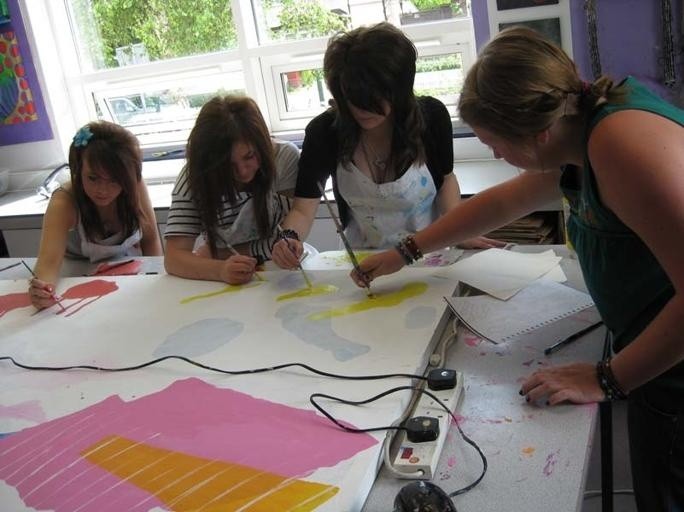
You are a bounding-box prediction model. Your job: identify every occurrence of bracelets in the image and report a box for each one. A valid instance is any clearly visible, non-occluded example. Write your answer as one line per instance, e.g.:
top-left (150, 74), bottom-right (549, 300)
top-left (597, 356), bottom-right (628, 403)
top-left (274, 229), bottom-right (300, 240)
top-left (395, 235), bottom-right (423, 266)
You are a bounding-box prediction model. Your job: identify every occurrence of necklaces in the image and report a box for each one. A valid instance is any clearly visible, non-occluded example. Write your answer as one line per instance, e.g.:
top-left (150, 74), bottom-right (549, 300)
top-left (368, 144), bottom-right (389, 172)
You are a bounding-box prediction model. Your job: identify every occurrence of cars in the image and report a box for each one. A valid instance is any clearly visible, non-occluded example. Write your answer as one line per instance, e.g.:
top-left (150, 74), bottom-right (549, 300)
top-left (108, 94), bottom-right (170, 120)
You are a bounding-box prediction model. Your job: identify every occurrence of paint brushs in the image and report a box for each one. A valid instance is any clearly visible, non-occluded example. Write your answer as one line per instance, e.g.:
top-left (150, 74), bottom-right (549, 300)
top-left (214, 229), bottom-right (261, 283)
top-left (317, 181), bottom-right (375, 300)
top-left (278, 225), bottom-right (314, 292)
top-left (82, 260), bottom-right (135, 276)
top-left (21, 260), bottom-right (68, 312)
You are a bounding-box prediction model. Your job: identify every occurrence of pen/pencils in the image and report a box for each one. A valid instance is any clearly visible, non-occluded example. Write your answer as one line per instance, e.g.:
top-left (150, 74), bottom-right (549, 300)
top-left (0, 255), bottom-right (23, 273)
top-left (544, 320), bottom-right (603, 355)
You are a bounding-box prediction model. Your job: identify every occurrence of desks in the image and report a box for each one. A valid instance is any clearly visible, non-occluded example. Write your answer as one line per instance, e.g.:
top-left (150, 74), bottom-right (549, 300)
top-left (1, 241), bottom-right (615, 510)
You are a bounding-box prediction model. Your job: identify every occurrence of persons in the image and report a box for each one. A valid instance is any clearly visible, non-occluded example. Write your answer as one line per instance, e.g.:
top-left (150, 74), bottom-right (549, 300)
top-left (163, 94), bottom-right (301, 284)
top-left (349, 24), bottom-right (684, 512)
top-left (271, 22), bottom-right (507, 269)
top-left (28, 120), bottom-right (163, 310)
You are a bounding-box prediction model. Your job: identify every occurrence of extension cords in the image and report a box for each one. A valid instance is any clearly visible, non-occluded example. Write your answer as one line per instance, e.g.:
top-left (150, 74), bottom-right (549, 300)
top-left (393, 370), bottom-right (465, 482)
